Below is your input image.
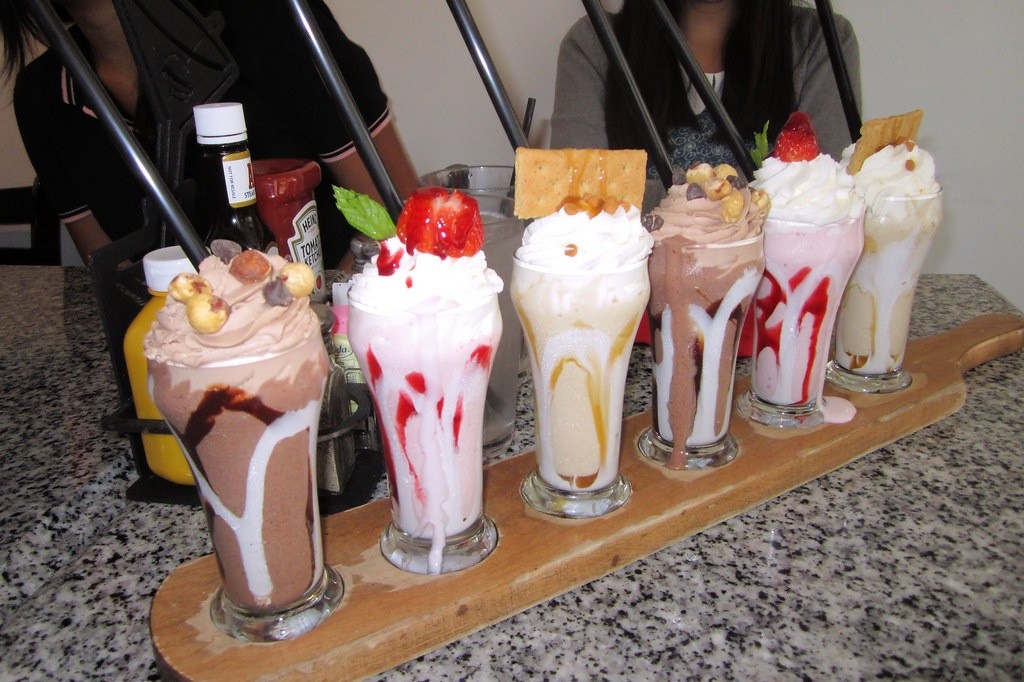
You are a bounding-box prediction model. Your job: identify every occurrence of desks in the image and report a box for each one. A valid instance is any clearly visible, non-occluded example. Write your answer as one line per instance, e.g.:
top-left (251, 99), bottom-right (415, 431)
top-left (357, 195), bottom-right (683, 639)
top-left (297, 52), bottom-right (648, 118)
top-left (0, 265), bottom-right (1024, 682)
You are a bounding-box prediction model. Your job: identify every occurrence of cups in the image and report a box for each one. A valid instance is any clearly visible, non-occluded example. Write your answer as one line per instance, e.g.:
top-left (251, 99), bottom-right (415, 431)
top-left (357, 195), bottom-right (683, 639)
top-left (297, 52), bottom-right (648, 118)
top-left (508, 244), bottom-right (651, 517)
top-left (735, 214), bottom-right (865, 429)
top-left (635, 231), bottom-right (766, 470)
top-left (347, 281), bottom-right (503, 575)
top-left (823, 185), bottom-right (948, 392)
top-left (243, 158), bottom-right (326, 305)
top-left (416, 164), bottom-right (520, 458)
top-left (143, 324), bottom-right (345, 642)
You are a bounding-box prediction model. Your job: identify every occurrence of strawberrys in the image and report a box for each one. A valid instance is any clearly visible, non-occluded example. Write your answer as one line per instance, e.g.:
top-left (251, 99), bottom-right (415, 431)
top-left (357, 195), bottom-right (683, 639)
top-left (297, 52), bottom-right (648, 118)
top-left (773, 111), bottom-right (820, 162)
top-left (396, 186), bottom-right (485, 257)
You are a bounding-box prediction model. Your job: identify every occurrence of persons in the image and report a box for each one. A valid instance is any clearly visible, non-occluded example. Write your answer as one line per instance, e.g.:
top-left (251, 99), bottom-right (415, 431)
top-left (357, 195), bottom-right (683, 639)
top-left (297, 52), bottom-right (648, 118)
top-left (550, 0), bottom-right (863, 220)
top-left (0, 0), bottom-right (421, 267)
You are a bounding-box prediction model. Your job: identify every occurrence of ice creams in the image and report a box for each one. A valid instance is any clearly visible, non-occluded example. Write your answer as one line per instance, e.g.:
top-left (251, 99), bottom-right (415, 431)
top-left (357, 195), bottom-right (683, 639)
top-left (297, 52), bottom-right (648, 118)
top-left (748, 153), bottom-right (866, 422)
top-left (839, 143), bottom-right (942, 375)
top-left (640, 163), bottom-right (771, 470)
top-left (348, 236), bottom-right (504, 576)
top-left (143, 237), bottom-right (328, 615)
top-left (509, 195), bottom-right (655, 491)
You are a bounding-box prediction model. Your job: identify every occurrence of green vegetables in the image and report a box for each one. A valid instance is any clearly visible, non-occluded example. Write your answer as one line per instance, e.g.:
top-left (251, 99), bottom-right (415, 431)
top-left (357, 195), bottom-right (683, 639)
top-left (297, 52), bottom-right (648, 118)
top-left (332, 184), bottom-right (397, 241)
top-left (750, 120), bottom-right (770, 169)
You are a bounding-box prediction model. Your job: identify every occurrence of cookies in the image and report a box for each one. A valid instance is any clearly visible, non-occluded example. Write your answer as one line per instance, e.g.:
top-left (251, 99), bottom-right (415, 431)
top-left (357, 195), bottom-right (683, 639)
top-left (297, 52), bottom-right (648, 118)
top-left (514, 147), bottom-right (648, 218)
top-left (847, 110), bottom-right (922, 174)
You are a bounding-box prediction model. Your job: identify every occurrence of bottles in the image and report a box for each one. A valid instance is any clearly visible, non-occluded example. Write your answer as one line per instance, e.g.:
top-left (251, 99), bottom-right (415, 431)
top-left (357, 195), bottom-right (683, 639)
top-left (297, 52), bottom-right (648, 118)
top-left (122, 242), bottom-right (211, 486)
top-left (181, 102), bottom-right (279, 265)
top-left (307, 307), bottom-right (381, 516)
